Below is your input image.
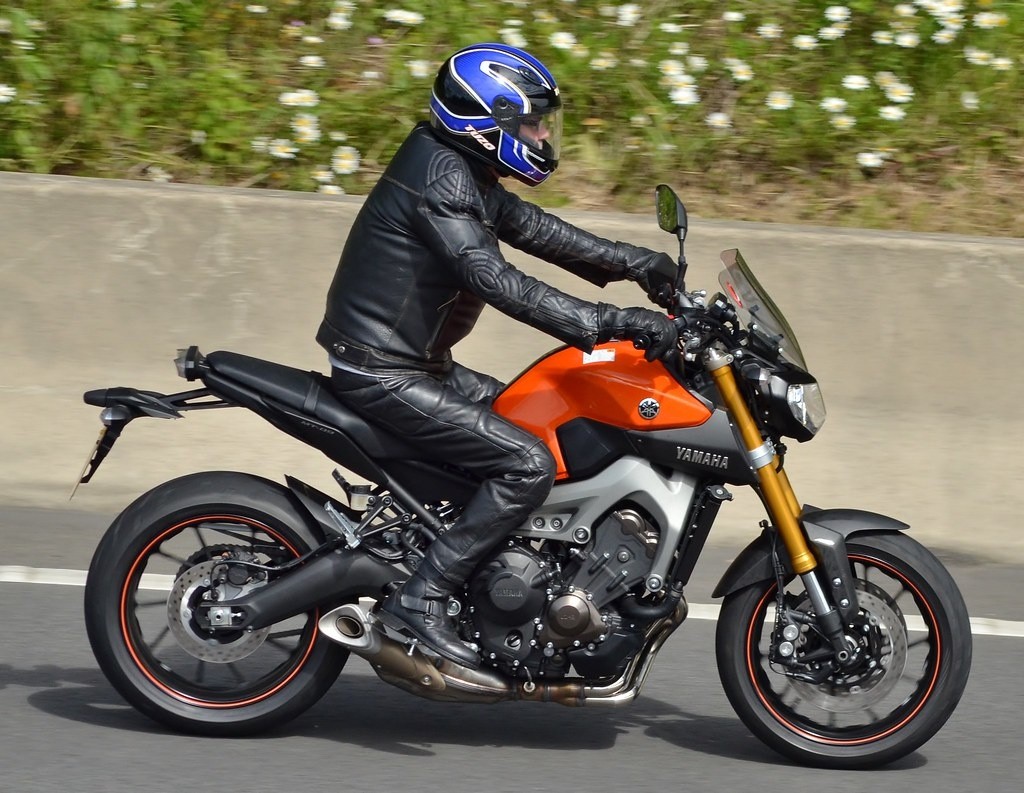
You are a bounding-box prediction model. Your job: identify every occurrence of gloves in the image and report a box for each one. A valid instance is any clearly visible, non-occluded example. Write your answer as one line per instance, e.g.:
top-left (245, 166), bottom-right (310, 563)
top-left (608, 241), bottom-right (686, 308)
top-left (596, 301), bottom-right (678, 363)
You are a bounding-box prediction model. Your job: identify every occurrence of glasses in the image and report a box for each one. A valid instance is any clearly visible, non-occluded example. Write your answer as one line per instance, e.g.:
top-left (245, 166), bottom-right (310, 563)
top-left (520, 116), bottom-right (552, 133)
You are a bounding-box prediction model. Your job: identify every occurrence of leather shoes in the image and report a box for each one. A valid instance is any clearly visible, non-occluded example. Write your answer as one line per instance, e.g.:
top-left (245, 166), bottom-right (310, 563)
top-left (370, 571), bottom-right (481, 670)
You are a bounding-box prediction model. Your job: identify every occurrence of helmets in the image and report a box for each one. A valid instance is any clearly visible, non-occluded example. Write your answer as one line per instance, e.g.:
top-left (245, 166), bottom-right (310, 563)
top-left (429, 43), bottom-right (563, 187)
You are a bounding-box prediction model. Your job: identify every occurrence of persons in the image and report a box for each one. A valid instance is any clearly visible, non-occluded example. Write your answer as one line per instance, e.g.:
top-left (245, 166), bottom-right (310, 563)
top-left (314, 42), bottom-right (686, 673)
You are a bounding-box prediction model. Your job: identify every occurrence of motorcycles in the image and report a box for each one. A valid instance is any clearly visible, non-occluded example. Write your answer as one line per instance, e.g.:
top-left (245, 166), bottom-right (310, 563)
top-left (68, 184), bottom-right (972, 771)
